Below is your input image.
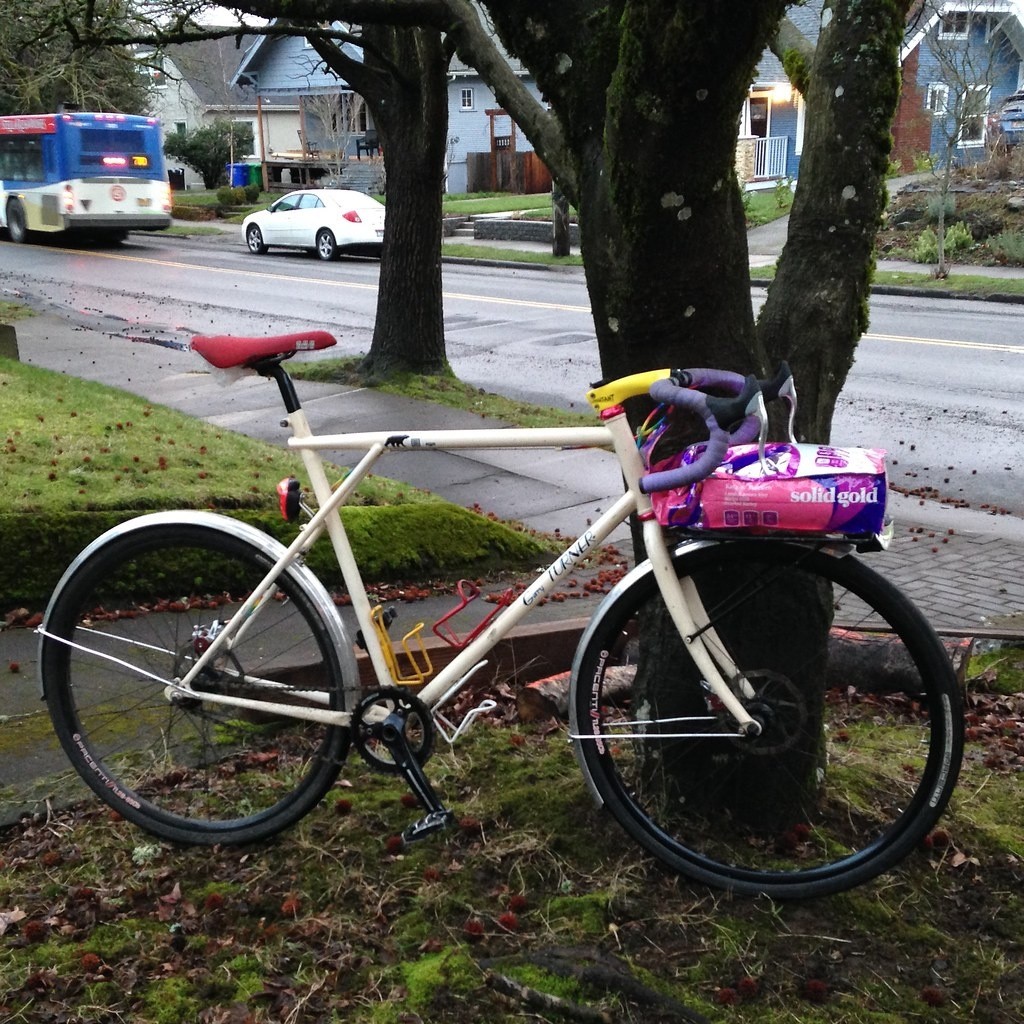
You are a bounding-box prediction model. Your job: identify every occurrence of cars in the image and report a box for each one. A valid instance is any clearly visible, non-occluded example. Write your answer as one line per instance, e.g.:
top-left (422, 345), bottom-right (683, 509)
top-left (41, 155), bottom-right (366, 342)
top-left (997, 86), bottom-right (1024, 149)
top-left (240, 188), bottom-right (387, 260)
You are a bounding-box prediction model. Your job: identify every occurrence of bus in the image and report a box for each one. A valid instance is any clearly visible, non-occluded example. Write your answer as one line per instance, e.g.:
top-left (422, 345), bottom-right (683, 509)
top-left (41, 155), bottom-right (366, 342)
top-left (0, 112), bottom-right (174, 247)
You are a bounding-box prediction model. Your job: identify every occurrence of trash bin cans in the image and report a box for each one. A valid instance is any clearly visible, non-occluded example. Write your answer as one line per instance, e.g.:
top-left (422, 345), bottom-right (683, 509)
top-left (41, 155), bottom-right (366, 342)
top-left (167, 168), bottom-right (185, 190)
top-left (248, 163), bottom-right (263, 192)
top-left (226, 163), bottom-right (250, 188)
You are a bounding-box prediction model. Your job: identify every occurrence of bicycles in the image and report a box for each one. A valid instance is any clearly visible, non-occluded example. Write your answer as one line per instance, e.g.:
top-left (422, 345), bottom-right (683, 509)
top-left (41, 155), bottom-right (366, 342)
top-left (33, 328), bottom-right (967, 902)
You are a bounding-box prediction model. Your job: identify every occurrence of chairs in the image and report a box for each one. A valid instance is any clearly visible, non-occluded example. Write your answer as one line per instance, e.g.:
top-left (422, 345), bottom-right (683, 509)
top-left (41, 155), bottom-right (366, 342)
top-left (298, 129), bottom-right (321, 161)
top-left (356, 129), bottom-right (381, 161)
